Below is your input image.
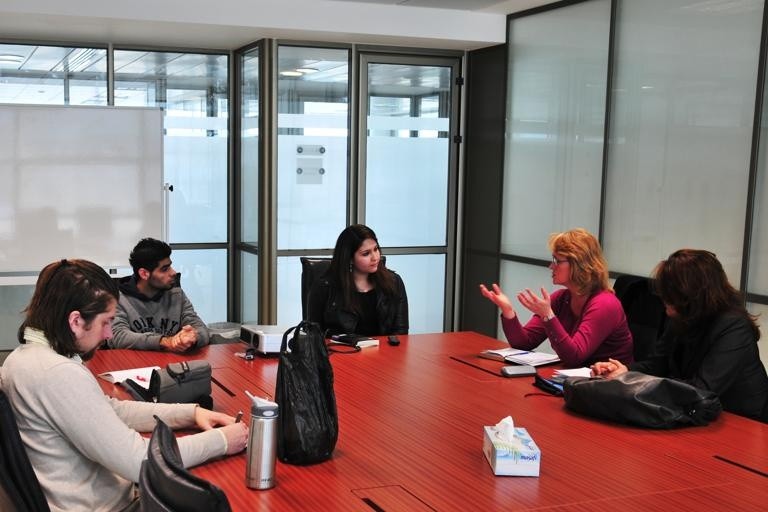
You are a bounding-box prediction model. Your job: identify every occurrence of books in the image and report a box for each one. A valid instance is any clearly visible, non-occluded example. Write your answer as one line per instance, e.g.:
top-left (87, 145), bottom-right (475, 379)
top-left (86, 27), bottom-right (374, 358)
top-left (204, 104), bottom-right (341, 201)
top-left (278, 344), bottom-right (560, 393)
top-left (96, 365), bottom-right (161, 391)
top-left (330, 329), bottom-right (380, 349)
top-left (552, 361), bottom-right (595, 380)
top-left (480, 345), bottom-right (561, 367)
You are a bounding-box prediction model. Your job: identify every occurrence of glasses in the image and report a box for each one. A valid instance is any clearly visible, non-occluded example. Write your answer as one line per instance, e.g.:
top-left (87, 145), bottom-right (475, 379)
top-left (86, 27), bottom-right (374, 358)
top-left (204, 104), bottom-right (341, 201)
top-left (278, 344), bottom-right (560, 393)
top-left (550, 256), bottom-right (569, 264)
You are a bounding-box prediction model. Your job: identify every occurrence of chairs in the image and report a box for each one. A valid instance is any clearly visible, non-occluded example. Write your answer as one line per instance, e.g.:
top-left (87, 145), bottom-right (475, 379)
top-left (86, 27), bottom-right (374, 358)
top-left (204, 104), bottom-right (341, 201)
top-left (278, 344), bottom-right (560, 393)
top-left (0, 390), bottom-right (51, 512)
top-left (139, 414), bottom-right (231, 512)
top-left (613, 273), bottom-right (670, 371)
top-left (109, 273), bottom-right (181, 288)
top-left (300, 257), bottom-right (387, 324)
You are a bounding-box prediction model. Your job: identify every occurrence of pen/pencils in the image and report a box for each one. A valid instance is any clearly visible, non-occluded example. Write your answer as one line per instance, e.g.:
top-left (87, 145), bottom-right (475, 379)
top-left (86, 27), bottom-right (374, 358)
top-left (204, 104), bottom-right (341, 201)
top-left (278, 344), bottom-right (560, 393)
top-left (235, 411), bottom-right (245, 424)
top-left (505, 352), bottom-right (528, 357)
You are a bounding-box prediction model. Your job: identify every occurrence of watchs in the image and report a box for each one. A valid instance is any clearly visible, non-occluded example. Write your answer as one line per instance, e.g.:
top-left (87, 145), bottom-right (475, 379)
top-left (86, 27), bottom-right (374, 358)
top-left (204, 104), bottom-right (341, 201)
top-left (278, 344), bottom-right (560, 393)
top-left (540, 313), bottom-right (555, 324)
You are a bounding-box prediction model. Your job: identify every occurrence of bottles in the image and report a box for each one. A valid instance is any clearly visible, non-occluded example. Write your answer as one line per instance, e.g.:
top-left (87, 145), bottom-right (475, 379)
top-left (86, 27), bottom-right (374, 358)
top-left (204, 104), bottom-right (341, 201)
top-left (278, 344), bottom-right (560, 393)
top-left (244, 401), bottom-right (280, 490)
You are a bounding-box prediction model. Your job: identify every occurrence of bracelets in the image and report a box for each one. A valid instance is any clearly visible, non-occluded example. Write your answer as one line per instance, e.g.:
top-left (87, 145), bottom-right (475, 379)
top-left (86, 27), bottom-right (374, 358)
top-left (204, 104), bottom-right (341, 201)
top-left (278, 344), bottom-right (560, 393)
top-left (217, 428), bottom-right (228, 454)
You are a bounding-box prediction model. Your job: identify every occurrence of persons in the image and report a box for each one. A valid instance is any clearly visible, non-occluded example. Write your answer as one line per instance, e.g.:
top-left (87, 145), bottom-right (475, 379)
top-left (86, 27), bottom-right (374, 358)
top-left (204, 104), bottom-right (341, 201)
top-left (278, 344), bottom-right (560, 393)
top-left (589, 248), bottom-right (768, 423)
top-left (106, 238), bottom-right (210, 352)
top-left (479, 228), bottom-right (635, 368)
top-left (308, 224), bottom-right (409, 334)
top-left (0, 258), bottom-right (251, 512)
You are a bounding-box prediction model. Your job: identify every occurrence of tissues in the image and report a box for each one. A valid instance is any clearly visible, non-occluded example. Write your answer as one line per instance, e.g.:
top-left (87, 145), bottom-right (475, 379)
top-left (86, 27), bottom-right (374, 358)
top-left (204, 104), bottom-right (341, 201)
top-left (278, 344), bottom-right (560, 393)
top-left (483, 416), bottom-right (542, 479)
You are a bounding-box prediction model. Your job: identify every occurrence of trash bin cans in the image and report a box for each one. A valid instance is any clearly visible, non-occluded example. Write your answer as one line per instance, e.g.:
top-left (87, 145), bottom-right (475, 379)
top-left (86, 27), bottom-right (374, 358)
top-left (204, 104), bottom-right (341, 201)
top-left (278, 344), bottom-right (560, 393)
top-left (207, 322), bottom-right (242, 343)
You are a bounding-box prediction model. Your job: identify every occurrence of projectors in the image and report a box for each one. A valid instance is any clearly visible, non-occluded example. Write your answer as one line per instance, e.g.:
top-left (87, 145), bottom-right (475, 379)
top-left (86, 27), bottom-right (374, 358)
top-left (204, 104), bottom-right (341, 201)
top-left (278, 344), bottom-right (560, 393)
top-left (239, 324), bottom-right (294, 355)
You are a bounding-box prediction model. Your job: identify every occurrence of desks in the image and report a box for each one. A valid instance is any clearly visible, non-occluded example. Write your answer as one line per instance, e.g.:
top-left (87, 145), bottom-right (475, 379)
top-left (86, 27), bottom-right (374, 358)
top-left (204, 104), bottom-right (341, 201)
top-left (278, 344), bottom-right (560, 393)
top-left (82, 330), bottom-right (768, 512)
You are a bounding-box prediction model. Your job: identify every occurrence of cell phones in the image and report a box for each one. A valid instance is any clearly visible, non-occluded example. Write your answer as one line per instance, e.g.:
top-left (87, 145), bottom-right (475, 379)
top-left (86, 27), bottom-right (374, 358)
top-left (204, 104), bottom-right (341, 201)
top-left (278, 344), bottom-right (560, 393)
top-left (388, 336), bottom-right (400, 346)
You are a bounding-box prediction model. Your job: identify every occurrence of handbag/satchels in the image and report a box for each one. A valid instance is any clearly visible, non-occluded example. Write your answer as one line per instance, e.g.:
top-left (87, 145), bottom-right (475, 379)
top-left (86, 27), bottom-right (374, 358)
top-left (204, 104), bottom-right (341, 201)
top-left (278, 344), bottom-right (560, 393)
top-left (146, 358), bottom-right (213, 409)
top-left (564, 370), bottom-right (722, 431)
